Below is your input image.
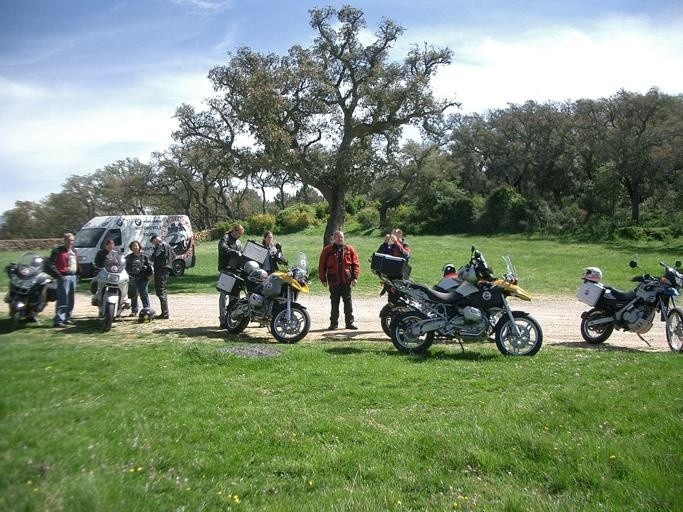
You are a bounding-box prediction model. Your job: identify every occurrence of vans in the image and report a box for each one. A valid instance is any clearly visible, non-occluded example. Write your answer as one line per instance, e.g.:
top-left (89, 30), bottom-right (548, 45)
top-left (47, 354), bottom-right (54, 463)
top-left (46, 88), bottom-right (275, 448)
top-left (73, 215), bottom-right (197, 281)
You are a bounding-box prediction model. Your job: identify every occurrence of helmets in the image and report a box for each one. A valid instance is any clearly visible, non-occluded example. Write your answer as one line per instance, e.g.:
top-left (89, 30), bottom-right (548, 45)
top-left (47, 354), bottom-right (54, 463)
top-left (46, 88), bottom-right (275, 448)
top-left (244, 261), bottom-right (260, 277)
top-left (441, 263), bottom-right (456, 281)
top-left (581, 267), bottom-right (603, 284)
top-left (248, 269), bottom-right (269, 285)
top-left (146, 265), bottom-right (155, 281)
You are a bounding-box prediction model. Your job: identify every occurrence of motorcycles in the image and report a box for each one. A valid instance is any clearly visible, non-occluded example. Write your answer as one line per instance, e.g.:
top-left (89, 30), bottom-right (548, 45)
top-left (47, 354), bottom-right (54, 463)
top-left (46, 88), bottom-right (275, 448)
top-left (2, 252), bottom-right (59, 327)
top-left (576, 257), bottom-right (683, 354)
top-left (88, 249), bottom-right (139, 332)
top-left (370, 247), bottom-right (544, 358)
top-left (214, 252), bottom-right (314, 344)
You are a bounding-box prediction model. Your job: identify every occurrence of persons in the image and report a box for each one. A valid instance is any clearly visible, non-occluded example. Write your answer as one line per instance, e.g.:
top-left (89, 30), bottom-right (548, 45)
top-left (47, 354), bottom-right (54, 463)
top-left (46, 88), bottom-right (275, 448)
top-left (258, 230), bottom-right (282, 328)
top-left (377, 228), bottom-right (411, 304)
top-left (94, 239), bottom-right (131, 310)
top-left (148, 235), bottom-right (177, 319)
top-left (217, 224), bottom-right (245, 329)
top-left (318, 230), bottom-right (360, 330)
top-left (124, 241), bottom-right (153, 317)
top-left (46, 232), bottom-right (81, 329)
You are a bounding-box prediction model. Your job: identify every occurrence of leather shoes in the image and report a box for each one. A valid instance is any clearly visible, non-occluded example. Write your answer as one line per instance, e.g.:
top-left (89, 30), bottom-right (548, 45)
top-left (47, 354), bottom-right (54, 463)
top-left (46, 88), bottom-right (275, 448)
top-left (329, 325), bottom-right (338, 330)
top-left (346, 325), bottom-right (358, 329)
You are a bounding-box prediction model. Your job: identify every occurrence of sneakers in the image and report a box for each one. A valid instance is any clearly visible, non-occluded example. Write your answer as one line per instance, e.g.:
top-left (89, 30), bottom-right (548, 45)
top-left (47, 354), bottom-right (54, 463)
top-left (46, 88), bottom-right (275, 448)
top-left (64, 320), bottom-right (76, 325)
top-left (154, 314), bottom-right (168, 319)
top-left (53, 322), bottom-right (66, 327)
top-left (129, 313), bottom-right (136, 317)
